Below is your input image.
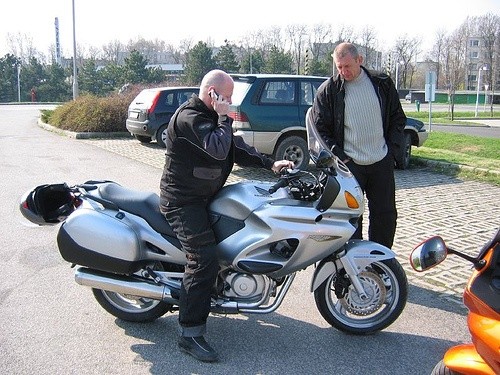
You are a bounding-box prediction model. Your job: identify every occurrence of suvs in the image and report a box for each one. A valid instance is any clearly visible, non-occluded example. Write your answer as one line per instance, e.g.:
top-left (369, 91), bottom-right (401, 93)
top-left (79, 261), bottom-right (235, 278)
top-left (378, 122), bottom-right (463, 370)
top-left (125, 86), bottom-right (201, 147)
top-left (217, 72), bottom-right (429, 169)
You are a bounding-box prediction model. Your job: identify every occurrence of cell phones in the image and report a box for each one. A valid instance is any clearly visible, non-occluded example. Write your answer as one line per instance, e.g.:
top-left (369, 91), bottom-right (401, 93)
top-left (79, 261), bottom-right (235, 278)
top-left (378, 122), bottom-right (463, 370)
top-left (208, 89), bottom-right (223, 101)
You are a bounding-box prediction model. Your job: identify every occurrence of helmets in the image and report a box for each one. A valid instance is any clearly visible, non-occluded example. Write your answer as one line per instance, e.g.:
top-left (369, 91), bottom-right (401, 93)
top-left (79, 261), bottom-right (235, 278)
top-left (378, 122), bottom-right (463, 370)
top-left (19, 181), bottom-right (82, 227)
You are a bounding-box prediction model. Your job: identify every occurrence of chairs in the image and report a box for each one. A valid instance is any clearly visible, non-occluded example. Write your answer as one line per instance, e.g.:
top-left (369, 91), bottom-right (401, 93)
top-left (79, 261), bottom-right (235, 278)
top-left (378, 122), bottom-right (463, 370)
top-left (263, 90), bottom-right (308, 104)
top-left (180, 95), bottom-right (187, 105)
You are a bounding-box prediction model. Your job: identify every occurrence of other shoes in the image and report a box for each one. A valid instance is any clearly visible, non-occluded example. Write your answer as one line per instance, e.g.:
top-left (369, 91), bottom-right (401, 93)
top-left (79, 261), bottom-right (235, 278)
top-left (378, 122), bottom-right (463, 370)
top-left (379, 273), bottom-right (391, 290)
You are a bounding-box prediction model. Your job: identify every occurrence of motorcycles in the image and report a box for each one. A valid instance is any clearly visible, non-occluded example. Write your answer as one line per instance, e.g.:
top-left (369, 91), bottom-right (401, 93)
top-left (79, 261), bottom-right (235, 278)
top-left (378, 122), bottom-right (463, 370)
top-left (18, 142), bottom-right (409, 334)
top-left (409, 222), bottom-right (500, 375)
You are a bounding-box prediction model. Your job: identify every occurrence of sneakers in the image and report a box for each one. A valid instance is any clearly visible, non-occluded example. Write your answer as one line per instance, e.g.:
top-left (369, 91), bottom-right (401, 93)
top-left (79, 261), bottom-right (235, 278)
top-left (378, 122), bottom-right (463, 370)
top-left (178, 335), bottom-right (219, 361)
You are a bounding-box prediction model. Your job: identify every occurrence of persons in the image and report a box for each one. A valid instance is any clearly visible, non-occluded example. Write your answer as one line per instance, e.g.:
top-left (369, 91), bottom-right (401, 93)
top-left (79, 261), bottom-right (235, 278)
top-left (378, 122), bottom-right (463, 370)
top-left (312, 42), bottom-right (407, 290)
top-left (159, 69), bottom-right (295, 362)
